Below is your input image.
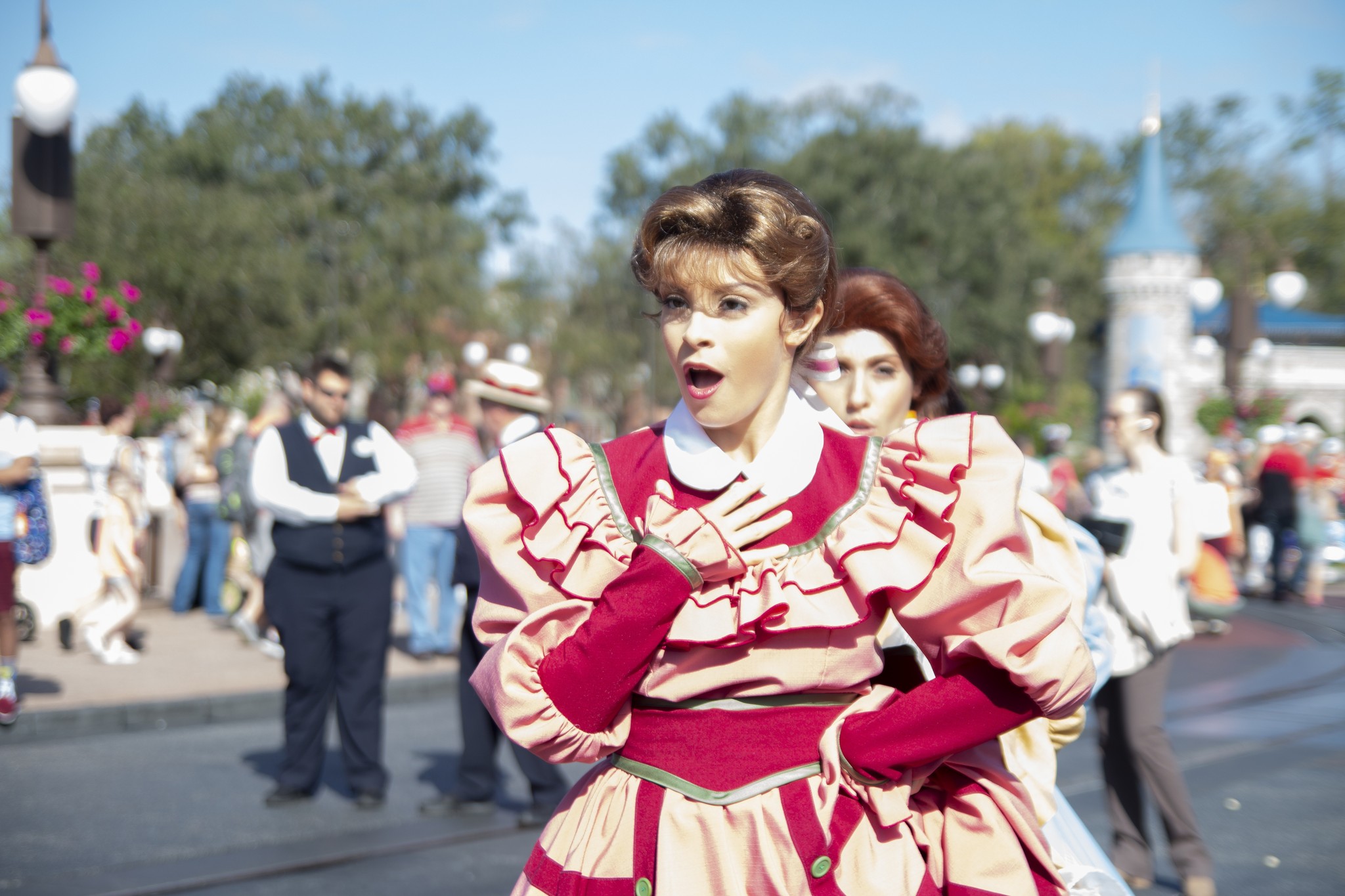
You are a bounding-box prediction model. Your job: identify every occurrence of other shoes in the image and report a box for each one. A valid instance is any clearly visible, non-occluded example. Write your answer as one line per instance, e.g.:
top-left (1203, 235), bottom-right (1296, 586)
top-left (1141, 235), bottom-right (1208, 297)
top-left (1, 695), bottom-right (20, 726)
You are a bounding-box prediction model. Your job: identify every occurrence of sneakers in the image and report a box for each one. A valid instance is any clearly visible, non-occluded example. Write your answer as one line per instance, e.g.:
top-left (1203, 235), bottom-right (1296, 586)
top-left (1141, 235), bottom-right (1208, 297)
top-left (415, 780), bottom-right (498, 821)
top-left (262, 781), bottom-right (311, 807)
top-left (516, 788), bottom-right (574, 827)
top-left (1111, 859), bottom-right (1158, 891)
top-left (1182, 873), bottom-right (1218, 896)
top-left (351, 779), bottom-right (385, 813)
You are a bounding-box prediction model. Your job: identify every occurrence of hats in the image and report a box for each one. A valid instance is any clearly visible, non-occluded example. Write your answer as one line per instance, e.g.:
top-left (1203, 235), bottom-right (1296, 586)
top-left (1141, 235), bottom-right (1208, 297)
top-left (465, 357), bottom-right (555, 421)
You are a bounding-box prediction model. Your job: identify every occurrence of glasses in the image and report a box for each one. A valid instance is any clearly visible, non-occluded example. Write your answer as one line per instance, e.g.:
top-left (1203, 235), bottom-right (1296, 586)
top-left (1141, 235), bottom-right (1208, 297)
top-left (311, 383), bottom-right (351, 400)
top-left (1097, 408), bottom-right (1155, 425)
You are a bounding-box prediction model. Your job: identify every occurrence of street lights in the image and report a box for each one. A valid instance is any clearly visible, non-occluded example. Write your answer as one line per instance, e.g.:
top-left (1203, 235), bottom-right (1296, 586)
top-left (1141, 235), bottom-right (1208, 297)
top-left (955, 344), bottom-right (1010, 422)
top-left (1190, 222), bottom-right (1299, 430)
top-left (1028, 293), bottom-right (1074, 458)
top-left (9, 0), bottom-right (79, 425)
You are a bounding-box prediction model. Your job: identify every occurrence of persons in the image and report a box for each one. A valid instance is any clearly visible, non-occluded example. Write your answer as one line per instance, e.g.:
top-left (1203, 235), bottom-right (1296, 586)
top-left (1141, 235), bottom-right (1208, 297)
top-left (1003, 398), bottom-right (1344, 636)
top-left (416, 355), bottom-right (573, 832)
top-left (0, 348), bottom-right (670, 726)
top-left (1068, 381), bottom-right (1217, 896)
top-left (461, 172), bottom-right (1095, 896)
top-left (249, 356), bottom-right (421, 804)
top-left (800, 271), bottom-right (1144, 896)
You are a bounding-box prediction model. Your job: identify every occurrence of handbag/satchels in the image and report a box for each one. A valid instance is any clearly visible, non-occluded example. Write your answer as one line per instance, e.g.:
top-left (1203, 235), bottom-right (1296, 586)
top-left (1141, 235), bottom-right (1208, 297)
top-left (11, 455), bottom-right (53, 566)
